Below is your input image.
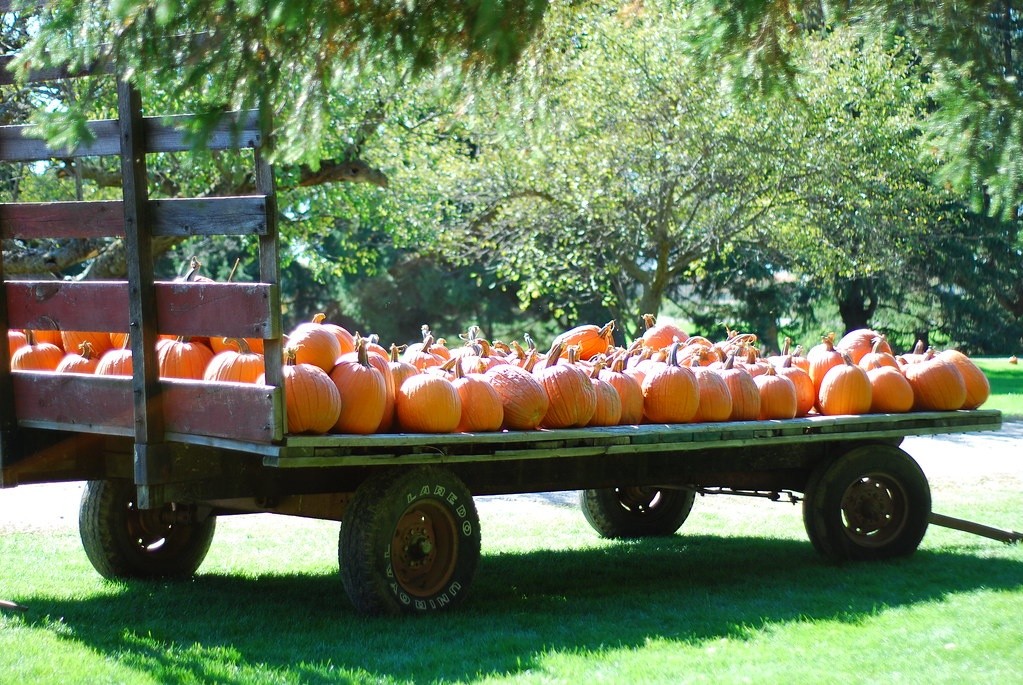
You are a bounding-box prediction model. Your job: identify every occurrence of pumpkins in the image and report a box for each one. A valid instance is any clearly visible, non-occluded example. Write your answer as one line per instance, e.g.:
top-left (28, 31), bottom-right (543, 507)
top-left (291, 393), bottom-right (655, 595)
top-left (8, 307), bottom-right (989, 434)
